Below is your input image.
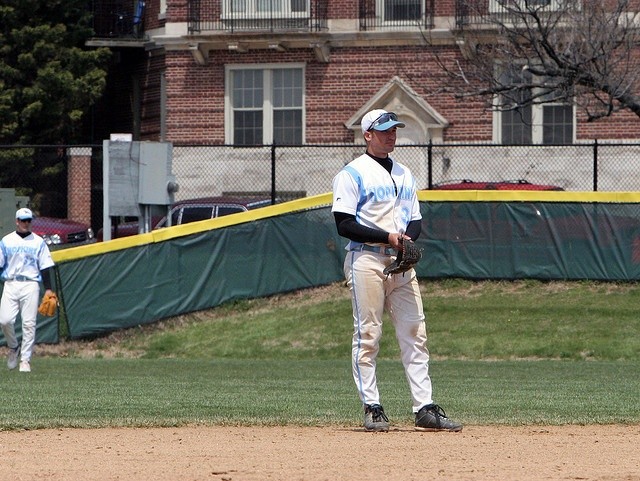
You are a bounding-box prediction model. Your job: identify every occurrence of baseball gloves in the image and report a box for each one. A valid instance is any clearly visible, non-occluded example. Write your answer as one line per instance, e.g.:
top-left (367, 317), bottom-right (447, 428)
top-left (38, 293), bottom-right (59, 317)
top-left (383, 234), bottom-right (421, 281)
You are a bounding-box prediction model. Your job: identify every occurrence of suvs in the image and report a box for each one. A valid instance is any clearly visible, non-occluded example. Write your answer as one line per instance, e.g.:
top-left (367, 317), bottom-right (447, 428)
top-left (420, 178), bottom-right (640, 265)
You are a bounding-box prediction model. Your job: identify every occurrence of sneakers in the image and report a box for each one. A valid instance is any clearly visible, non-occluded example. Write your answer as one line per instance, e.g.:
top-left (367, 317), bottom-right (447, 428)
top-left (7, 346), bottom-right (20, 369)
top-left (364, 404), bottom-right (388, 432)
top-left (19, 362), bottom-right (31, 372)
top-left (415, 404), bottom-right (463, 431)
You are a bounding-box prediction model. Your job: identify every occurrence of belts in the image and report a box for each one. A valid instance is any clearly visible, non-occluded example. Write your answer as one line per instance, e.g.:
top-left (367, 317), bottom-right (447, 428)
top-left (360, 244), bottom-right (398, 255)
top-left (12, 276), bottom-right (29, 281)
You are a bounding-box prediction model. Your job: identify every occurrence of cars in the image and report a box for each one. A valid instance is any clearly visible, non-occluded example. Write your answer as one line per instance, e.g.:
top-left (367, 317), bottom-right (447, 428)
top-left (98, 197), bottom-right (341, 265)
top-left (30, 217), bottom-right (98, 254)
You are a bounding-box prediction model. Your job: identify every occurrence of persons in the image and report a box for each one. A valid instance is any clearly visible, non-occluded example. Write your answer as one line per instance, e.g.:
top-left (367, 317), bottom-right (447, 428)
top-left (0, 208), bottom-right (59, 373)
top-left (331, 107), bottom-right (462, 431)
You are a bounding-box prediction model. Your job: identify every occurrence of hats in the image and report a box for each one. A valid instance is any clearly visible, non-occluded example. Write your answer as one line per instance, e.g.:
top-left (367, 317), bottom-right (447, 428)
top-left (15, 207), bottom-right (33, 219)
top-left (361, 109), bottom-right (405, 135)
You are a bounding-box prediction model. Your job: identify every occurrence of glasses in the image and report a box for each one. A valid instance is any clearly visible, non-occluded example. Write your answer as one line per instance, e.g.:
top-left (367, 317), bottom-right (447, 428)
top-left (368, 112), bottom-right (398, 131)
top-left (20, 218), bottom-right (32, 223)
